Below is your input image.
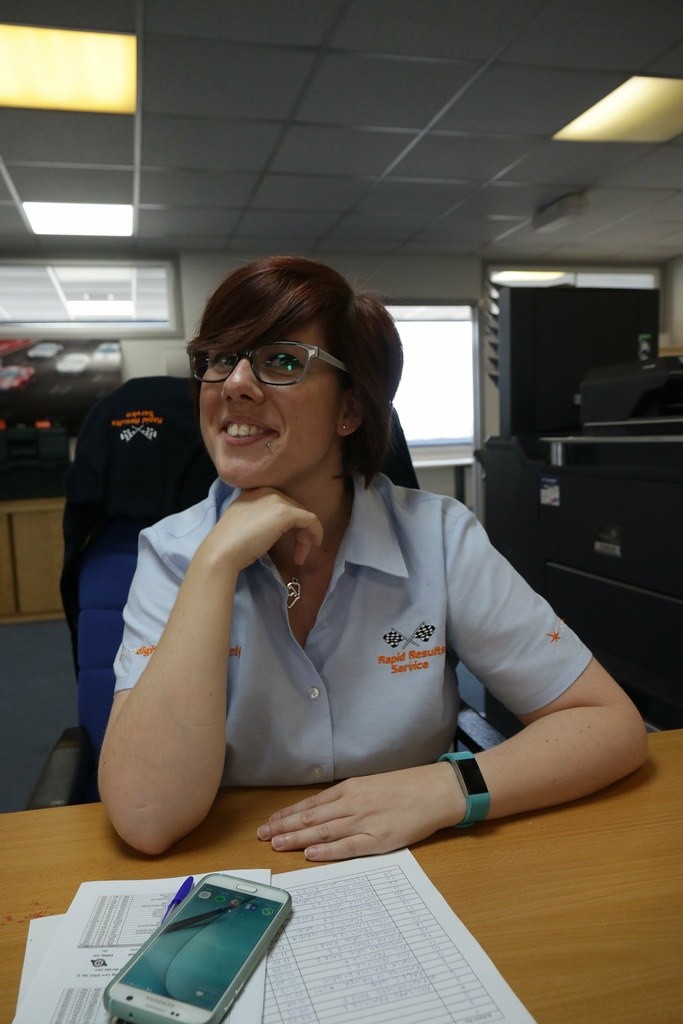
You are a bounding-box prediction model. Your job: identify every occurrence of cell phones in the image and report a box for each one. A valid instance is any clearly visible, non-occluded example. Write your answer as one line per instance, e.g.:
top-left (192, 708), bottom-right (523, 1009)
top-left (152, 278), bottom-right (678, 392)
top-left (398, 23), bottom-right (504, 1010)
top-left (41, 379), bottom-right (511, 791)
top-left (101, 873), bottom-right (293, 1024)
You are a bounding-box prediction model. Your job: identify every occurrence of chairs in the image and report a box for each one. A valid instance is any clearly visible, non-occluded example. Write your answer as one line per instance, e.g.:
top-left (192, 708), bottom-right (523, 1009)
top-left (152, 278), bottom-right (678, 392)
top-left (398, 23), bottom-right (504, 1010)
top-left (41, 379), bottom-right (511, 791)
top-left (24, 377), bottom-right (508, 809)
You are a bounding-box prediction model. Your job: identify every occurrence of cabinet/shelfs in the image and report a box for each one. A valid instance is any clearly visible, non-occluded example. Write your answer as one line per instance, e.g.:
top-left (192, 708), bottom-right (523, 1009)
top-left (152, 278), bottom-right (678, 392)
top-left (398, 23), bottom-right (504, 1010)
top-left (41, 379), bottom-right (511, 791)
top-left (0, 497), bottom-right (68, 626)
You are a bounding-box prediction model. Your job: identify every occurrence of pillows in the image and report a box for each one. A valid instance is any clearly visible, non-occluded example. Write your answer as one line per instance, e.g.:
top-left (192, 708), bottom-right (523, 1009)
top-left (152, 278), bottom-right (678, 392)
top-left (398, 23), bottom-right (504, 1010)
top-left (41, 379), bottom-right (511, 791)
top-left (76, 551), bottom-right (136, 749)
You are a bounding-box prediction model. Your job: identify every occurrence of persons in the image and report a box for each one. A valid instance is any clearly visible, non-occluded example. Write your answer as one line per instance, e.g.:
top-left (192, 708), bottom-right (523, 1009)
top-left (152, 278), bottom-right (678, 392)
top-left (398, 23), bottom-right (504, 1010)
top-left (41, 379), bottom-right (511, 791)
top-left (98, 257), bottom-right (649, 861)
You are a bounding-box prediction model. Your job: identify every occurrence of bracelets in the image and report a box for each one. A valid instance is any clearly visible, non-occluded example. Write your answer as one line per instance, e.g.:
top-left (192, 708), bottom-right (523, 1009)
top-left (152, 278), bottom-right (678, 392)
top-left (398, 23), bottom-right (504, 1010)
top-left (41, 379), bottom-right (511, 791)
top-left (440, 751), bottom-right (491, 828)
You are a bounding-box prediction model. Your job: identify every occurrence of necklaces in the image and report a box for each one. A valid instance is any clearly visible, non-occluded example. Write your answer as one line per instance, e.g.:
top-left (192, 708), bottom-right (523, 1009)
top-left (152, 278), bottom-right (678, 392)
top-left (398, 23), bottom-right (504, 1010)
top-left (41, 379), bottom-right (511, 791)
top-left (284, 570), bottom-right (302, 609)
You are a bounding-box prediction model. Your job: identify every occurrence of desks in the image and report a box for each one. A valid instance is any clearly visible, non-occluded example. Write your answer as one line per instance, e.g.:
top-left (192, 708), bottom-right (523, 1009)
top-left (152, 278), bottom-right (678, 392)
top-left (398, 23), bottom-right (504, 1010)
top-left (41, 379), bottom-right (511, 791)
top-left (0, 727), bottom-right (683, 1024)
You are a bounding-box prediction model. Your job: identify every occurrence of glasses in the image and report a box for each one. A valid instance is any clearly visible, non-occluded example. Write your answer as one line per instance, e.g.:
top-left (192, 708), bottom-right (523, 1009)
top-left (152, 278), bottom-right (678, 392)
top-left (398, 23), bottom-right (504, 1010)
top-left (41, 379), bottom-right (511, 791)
top-left (184, 335), bottom-right (346, 385)
